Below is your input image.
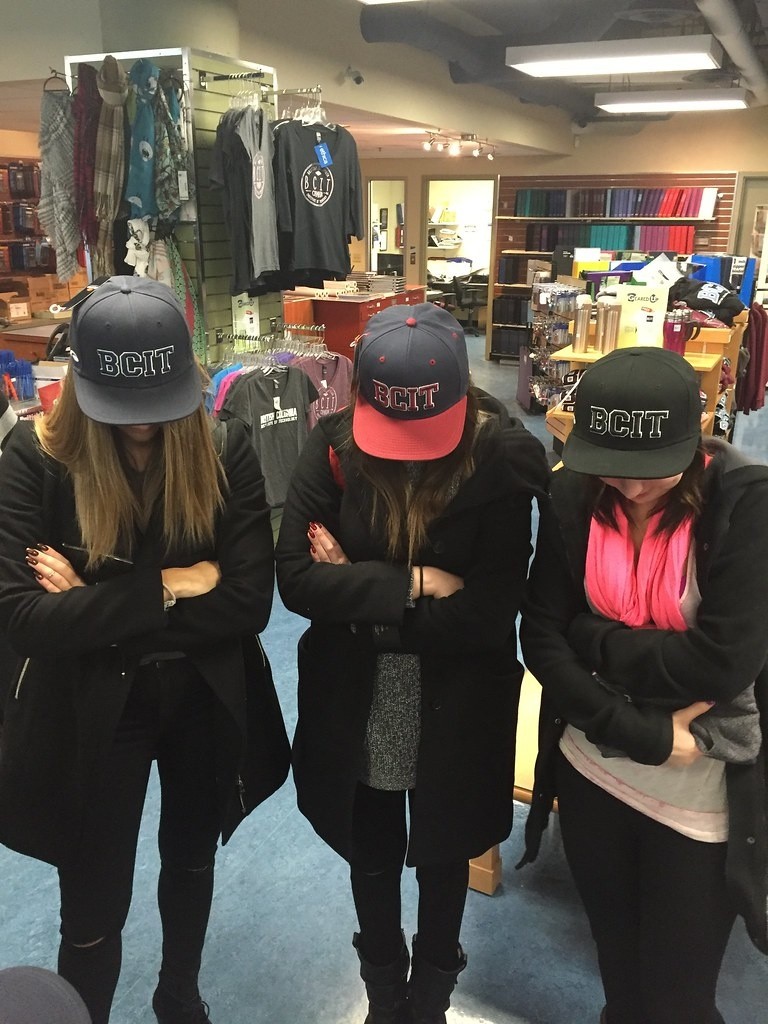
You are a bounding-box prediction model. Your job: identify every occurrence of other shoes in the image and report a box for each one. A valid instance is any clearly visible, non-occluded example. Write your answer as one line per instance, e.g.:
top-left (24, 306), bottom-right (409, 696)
top-left (152, 982), bottom-right (213, 1024)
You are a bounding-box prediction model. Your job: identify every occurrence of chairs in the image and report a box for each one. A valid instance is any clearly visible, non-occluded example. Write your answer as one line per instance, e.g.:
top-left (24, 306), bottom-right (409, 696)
top-left (454, 277), bottom-right (487, 337)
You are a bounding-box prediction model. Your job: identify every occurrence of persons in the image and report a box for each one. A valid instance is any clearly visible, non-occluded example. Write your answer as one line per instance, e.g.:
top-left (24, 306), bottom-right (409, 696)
top-left (0, 275), bottom-right (291, 1024)
top-left (272, 303), bottom-right (551, 1024)
top-left (517, 346), bottom-right (768, 1024)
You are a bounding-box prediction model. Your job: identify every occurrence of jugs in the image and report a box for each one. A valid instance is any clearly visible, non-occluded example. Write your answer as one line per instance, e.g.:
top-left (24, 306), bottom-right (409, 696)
top-left (663, 309), bottom-right (701, 357)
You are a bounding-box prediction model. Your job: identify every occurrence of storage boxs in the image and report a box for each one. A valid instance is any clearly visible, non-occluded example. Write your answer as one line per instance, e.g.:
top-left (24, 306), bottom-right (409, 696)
top-left (0, 270), bottom-right (88, 322)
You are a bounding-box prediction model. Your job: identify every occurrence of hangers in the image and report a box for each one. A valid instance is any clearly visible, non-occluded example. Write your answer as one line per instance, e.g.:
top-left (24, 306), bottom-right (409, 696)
top-left (43, 71), bottom-right (69, 91)
top-left (209, 322), bottom-right (339, 377)
top-left (227, 71), bottom-right (335, 131)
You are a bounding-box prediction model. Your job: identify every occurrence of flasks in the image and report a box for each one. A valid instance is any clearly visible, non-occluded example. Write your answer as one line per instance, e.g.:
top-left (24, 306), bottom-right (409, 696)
top-left (594, 302), bottom-right (622, 355)
top-left (573, 302), bottom-right (591, 353)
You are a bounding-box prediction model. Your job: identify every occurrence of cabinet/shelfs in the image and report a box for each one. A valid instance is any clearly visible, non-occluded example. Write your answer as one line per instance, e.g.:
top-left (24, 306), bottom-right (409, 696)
top-left (312, 285), bottom-right (430, 360)
top-left (428, 223), bottom-right (461, 249)
top-left (484, 217), bottom-right (748, 444)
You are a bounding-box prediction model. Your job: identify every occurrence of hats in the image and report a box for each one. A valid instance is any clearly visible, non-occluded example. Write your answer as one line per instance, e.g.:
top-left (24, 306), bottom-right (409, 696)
top-left (353, 302), bottom-right (469, 461)
top-left (561, 346), bottom-right (702, 479)
top-left (68, 276), bottom-right (201, 425)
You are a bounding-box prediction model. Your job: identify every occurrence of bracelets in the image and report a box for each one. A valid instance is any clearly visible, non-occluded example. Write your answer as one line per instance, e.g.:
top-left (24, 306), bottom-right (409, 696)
top-left (419, 565), bottom-right (424, 597)
top-left (163, 583), bottom-right (176, 608)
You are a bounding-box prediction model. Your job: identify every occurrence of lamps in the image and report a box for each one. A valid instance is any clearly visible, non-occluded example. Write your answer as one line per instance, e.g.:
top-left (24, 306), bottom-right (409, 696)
top-left (594, 63), bottom-right (752, 114)
top-left (472, 143), bottom-right (485, 157)
top-left (505, 35), bottom-right (725, 78)
top-left (450, 143), bottom-right (463, 155)
top-left (422, 133), bottom-right (436, 151)
top-left (437, 140), bottom-right (449, 151)
top-left (487, 147), bottom-right (495, 160)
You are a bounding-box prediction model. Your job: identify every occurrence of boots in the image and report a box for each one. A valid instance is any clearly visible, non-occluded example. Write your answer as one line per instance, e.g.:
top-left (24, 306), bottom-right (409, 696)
top-left (408, 932), bottom-right (468, 1024)
top-left (353, 928), bottom-right (409, 1023)
